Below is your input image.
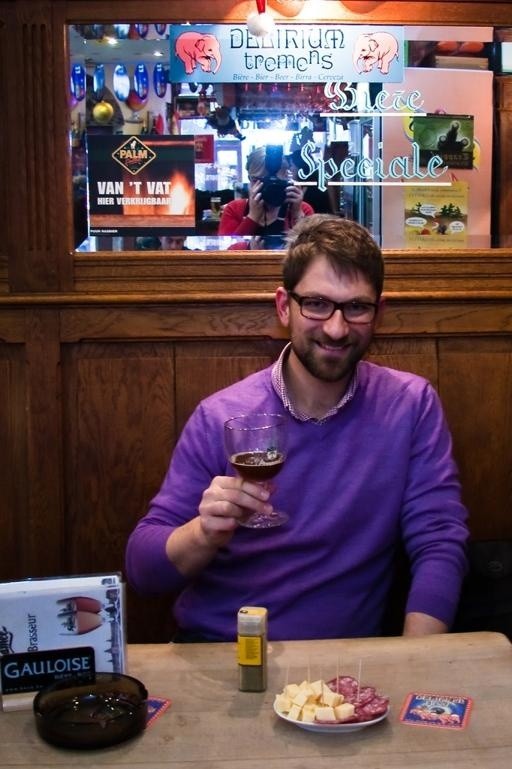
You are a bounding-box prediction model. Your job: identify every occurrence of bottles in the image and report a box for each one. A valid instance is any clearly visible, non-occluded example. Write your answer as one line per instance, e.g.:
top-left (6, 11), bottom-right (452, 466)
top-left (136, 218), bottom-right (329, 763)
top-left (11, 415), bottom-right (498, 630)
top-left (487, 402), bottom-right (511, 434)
top-left (238, 607), bottom-right (270, 692)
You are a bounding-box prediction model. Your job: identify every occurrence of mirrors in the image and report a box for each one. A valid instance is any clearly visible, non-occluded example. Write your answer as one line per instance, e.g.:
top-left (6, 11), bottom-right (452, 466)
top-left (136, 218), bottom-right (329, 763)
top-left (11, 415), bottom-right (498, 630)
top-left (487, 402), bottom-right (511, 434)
top-left (1, 17), bottom-right (512, 293)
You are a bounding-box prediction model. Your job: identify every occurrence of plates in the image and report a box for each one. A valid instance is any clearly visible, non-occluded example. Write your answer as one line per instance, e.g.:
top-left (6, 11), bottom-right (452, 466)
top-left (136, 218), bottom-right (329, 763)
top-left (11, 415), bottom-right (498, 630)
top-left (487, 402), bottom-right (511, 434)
top-left (272, 698), bottom-right (390, 734)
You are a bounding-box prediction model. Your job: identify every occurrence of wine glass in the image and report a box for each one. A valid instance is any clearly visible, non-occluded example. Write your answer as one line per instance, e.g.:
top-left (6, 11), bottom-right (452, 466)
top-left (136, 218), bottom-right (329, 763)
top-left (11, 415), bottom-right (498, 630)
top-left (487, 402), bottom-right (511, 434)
top-left (221, 412), bottom-right (291, 530)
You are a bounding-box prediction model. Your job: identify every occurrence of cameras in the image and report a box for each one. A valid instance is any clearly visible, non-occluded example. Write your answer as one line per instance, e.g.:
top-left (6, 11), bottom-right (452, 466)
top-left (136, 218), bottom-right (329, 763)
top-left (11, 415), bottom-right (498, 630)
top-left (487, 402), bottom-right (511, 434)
top-left (252, 142), bottom-right (294, 211)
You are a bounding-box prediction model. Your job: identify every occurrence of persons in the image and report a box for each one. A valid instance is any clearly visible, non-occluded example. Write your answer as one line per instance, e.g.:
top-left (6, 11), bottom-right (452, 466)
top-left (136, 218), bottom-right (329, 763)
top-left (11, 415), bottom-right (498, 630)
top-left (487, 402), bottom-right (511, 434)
top-left (290, 149), bottom-right (333, 215)
top-left (226, 236), bottom-right (288, 250)
top-left (139, 235), bottom-right (201, 250)
top-left (218, 146), bottom-right (315, 236)
top-left (125, 213), bottom-right (470, 637)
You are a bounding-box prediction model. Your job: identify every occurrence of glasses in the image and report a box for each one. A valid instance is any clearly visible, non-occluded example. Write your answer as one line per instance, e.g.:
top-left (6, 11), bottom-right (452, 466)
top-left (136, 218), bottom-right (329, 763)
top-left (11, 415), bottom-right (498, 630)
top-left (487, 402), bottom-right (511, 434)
top-left (288, 291), bottom-right (379, 324)
